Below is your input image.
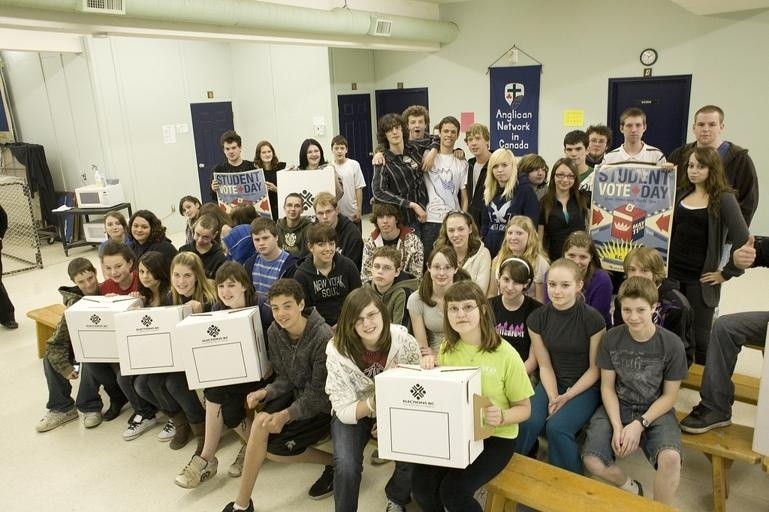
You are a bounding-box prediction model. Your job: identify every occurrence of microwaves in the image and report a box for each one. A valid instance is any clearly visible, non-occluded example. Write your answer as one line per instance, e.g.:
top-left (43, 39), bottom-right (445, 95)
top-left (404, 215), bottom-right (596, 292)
top-left (74, 183), bottom-right (124, 209)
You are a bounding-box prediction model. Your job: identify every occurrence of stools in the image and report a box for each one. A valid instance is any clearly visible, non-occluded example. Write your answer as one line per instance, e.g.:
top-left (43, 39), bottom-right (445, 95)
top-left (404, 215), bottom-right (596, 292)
top-left (25, 303), bottom-right (67, 359)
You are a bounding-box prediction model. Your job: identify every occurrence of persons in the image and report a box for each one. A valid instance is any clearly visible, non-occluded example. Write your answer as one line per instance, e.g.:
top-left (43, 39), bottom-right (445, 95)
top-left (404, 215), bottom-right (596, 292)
top-left (0, 204), bottom-right (19, 329)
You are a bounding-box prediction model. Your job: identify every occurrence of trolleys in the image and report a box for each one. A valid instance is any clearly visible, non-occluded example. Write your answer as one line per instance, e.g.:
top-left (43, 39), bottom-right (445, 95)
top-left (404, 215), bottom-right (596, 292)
top-left (33, 222), bottom-right (58, 247)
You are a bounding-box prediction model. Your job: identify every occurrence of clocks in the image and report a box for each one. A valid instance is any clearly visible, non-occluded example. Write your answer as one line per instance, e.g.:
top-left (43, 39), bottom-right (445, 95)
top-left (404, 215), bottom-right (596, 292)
top-left (640, 48), bottom-right (658, 66)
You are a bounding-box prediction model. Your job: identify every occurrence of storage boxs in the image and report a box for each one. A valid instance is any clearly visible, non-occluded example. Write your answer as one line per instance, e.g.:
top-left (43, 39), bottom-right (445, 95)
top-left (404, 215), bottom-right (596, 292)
top-left (115, 304), bottom-right (192, 377)
top-left (372, 361), bottom-right (484, 471)
top-left (173, 305), bottom-right (270, 390)
top-left (63, 294), bottom-right (145, 365)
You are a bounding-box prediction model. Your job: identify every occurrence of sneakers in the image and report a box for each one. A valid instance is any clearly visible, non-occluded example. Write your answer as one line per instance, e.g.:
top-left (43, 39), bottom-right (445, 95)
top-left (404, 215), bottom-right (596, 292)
top-left (174, 453), bottom-right (218, 489)
top-left (1, 318), bottom-right (19, 328)
top-left (369, 448), bottom-right (393, 466)
top-left (228, 442), bottom-right (249, 477)
top-left (38, 405), bottom-right (102, 432)
top-left (121, 414), bottom-right (157, 441)
top-left (222, 496), bottom-right (255, 512)
top-left (307, 463), bottom-right (335, 500)
top-left (157, 416), bottom-right (177, 442)
top-left (679, 399), bottom-right (733, 435)
top-left (386, 499), bottom-right (407, 512)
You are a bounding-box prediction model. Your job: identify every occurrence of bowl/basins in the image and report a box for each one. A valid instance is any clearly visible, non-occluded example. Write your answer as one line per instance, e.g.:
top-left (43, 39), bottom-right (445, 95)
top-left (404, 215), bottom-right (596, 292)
top-left (106, 178), bottom-right (119, 185)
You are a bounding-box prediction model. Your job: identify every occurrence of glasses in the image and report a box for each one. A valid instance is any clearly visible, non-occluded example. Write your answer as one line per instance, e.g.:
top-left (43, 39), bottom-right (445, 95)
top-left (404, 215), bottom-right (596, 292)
top-left (372, 264), bottom-right (395, 271)
top-left (555, 173), bottom-right (576, 180)
top-left (446, 305), bottom-right (478, 314)
top-left (432, 265), bottom-right (453, 272)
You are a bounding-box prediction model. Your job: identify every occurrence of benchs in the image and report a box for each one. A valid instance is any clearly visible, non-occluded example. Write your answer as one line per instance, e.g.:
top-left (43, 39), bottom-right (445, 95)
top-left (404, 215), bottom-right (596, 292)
top-left (472, 451), bottom-right (680, 510)
top-left (673, 410), bottom-right (768, 510)
top-left (680, 363), bottom-right (762, 406)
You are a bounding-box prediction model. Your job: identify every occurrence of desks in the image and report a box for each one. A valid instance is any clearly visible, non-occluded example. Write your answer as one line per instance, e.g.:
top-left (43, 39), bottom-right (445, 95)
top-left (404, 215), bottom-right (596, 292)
top-left (53, 203), bottom-right (133, 256)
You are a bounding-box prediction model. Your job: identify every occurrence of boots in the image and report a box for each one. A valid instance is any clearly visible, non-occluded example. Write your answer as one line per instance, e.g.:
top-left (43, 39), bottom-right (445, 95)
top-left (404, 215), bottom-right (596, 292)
top-left (183, 421), bottom-right (205, 474)
top-left (169, 409), bottom-right (190, 450)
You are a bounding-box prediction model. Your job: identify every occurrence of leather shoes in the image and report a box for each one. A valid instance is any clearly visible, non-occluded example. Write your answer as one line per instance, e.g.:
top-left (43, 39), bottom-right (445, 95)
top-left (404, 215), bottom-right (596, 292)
top-left (102, 399), bottom-right (132, 422)
top-left (633, 479), bottom-right (645, 497)
